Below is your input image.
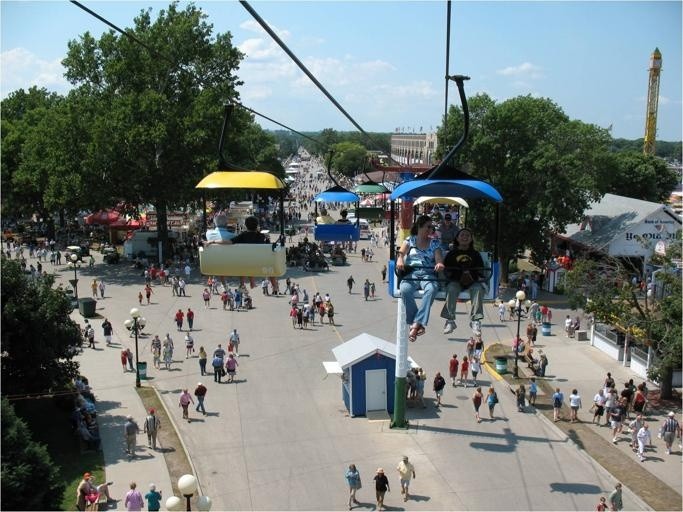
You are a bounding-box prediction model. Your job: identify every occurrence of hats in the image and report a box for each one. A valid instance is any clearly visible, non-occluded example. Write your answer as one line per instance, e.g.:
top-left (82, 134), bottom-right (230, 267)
top-left (376, 469), bottom-right (385, 474)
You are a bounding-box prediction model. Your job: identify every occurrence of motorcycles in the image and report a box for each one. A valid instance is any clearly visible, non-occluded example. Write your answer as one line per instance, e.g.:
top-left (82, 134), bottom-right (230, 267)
top-left (299, 245), bottom-right (339, 273)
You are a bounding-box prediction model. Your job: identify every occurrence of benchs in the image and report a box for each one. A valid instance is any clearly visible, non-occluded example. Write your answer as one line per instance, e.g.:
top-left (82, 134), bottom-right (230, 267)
top-left (75, 489), bottom-right (99, 512)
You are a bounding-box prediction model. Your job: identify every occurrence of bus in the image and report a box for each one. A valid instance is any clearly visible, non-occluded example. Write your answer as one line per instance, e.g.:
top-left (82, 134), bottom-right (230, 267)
top-left (347, 212), bottom-right (370, 240)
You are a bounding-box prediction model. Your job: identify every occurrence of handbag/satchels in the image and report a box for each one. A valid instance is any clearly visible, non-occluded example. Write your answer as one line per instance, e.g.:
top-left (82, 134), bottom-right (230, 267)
top-left (492, 392), bottom-right (498, 403)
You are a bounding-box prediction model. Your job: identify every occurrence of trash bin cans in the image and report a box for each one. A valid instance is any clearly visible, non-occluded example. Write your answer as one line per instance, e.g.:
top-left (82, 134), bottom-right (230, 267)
top-left (495, 357), bottom-right (507, 374)
top-left (542, 322), bottom-right (551, 336)
top-left (135, 362), bottom-right (147, 380)
top-left (78, 297), bottom-right (97, 318)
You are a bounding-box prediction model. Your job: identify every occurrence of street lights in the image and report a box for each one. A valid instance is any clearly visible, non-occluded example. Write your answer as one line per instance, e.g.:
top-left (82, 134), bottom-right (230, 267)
top-left (507, 290), bottom-right (532, 380)
top-left (67, 254), bottom-right (83, 302)
top-left (123, 307), bottom-right (147, 387)
top-left (166, 473), bottom-right (214, 511)
